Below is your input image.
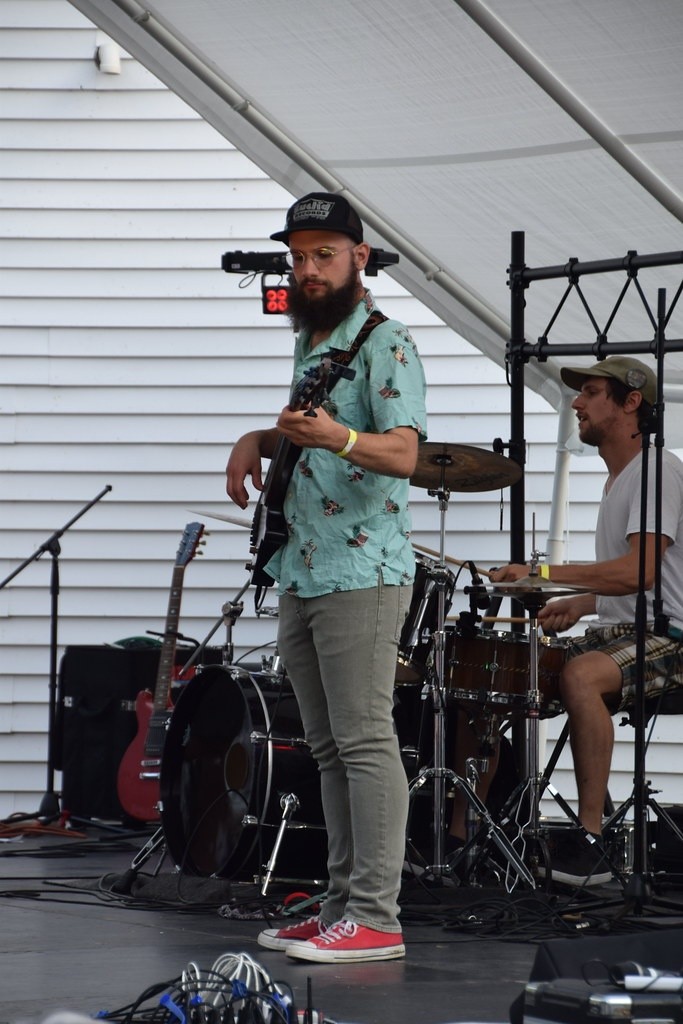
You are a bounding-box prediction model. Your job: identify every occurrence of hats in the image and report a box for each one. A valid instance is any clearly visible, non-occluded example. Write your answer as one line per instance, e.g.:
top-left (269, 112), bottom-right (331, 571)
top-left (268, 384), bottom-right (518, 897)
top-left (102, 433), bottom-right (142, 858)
top-left (270, 193), bottom-right (363, 245)
top-left (561, 357), bottom-right (656, 406)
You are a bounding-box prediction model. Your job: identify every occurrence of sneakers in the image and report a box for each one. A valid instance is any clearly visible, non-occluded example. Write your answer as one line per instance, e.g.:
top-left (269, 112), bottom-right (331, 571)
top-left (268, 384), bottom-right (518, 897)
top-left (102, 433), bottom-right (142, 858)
top-left (538, 839), bottom-right (612, 887)
top-left (257, 916), bottom-right (332, 951)
top-left (286, 919), bottom-right (405, 963)
top-left (402, 840), bottom-right (467, 887)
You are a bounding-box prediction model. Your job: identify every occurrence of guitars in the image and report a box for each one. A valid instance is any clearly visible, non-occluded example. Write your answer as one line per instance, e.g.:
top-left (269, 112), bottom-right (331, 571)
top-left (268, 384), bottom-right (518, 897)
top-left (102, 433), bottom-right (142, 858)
top-left (245, 358), bottom-right (356, 588)
top-left (115, 521), bottom-right (210, 820)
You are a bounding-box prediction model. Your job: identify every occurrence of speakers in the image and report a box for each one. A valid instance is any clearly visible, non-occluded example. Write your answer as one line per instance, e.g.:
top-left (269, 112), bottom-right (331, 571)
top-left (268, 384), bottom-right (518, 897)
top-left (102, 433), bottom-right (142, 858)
top-left (60, 644), bottom-right (224, 821)
top-left (530, 932), bottom-right (683, 983)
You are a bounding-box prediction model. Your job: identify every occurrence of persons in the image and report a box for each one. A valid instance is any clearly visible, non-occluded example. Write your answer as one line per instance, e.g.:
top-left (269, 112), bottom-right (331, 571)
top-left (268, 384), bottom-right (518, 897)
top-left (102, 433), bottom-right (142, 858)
top-left (224, 191), bottom-right (428, 964)
top-left (401, 356), bottom-right (683, 888)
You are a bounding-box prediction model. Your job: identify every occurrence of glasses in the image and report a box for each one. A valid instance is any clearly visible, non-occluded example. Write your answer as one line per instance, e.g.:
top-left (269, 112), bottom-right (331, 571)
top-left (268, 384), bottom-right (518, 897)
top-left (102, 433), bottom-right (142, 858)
top-left (286, 247), bottom-right (354, 268)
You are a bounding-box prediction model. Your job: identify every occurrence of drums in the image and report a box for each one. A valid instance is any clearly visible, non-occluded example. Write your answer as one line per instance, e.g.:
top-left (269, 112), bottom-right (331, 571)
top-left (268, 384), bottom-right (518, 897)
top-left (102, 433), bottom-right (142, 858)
top-left (158, 550), bottom-right (566, 887)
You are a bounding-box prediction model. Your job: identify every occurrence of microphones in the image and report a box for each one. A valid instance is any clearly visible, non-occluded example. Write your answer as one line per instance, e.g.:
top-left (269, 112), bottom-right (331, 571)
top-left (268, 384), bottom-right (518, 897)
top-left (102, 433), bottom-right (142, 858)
top-left (466, 558), bottom-right (491, 610)
top-left (610, 959), bottom-right (683, 987)
top-left (659, 623), bottom-right (683, 640)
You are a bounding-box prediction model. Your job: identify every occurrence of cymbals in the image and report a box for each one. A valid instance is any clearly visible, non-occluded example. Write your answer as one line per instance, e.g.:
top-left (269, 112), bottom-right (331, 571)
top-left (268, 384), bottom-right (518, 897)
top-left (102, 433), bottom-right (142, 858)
top-left (470, 575), bottom-right (598, 603)
top-left (410, 440), bottom-right (522, 493)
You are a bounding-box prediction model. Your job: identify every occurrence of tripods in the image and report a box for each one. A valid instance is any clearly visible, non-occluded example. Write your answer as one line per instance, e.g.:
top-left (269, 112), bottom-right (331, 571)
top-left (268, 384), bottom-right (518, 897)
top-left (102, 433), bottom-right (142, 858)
top-left (0, 486), bottom-right (133, 839)
top-left (406, 288), bottom-right (683, 933)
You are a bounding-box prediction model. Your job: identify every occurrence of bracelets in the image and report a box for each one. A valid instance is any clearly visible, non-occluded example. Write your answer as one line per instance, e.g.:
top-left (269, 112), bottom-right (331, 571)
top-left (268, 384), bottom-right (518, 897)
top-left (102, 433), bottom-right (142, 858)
top-left (335, 427), bottom-right (357, 457)
top-left (541, 563), bottom-right (550, 580)
